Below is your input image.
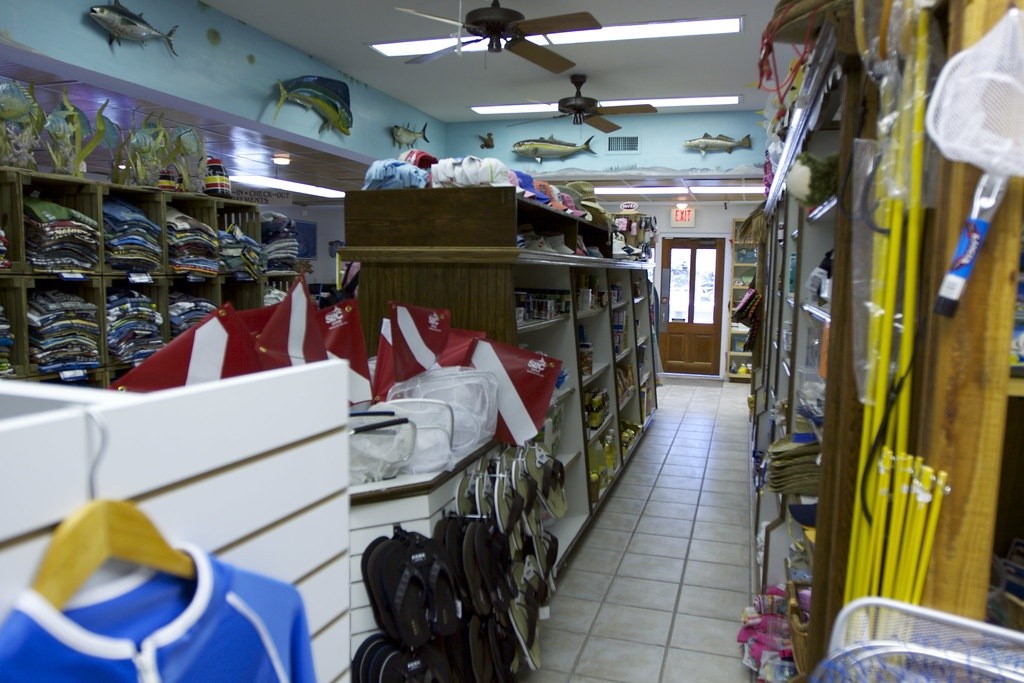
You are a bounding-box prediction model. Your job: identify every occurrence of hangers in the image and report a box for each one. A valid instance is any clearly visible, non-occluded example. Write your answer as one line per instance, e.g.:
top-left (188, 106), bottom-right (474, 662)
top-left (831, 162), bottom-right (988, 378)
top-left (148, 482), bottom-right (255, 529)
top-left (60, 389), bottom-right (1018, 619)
top-left (1, 404), bottom-right (302, 683)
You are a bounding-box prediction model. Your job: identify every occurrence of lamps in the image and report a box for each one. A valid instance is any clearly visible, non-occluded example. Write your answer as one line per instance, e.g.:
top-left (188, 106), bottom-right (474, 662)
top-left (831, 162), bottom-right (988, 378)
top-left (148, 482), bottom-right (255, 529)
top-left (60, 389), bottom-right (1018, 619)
top-left (225, 173), bottom-right (346, 200)
top-left (466, 93), bottom-right (740, 116)
top-left (365, 14), bottom-right (746, 60)
top-left (269, 156), bottom-right (293, 165)
top-left (593, 186), bottom-right (766, 197)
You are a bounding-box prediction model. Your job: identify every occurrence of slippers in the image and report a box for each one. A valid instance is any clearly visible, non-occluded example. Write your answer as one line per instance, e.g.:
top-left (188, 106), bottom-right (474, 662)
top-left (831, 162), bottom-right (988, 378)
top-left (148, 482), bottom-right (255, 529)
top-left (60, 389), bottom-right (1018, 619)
top-left (350, 439), bottom-right (569, 683)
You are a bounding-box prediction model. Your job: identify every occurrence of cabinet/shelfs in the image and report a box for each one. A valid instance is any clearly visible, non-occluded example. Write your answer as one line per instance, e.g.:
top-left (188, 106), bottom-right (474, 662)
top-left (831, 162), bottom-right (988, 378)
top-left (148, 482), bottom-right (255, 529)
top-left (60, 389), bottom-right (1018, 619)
top-left (344, 185), bottom-right (661, 587)
top-left (2, 164), bottom-right (305, 385)
top-left (742, 1), bottom-right (1024, 681)
top-left (724, 217), bottom-right (761, 382)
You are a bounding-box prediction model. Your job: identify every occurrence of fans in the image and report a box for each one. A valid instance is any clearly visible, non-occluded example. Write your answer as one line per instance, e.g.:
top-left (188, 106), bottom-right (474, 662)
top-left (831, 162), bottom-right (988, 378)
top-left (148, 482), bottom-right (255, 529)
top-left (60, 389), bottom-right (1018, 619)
top-left (503, 72), bottom-right (659, 135)
top-left (392, 0), bottom-right (602, 74)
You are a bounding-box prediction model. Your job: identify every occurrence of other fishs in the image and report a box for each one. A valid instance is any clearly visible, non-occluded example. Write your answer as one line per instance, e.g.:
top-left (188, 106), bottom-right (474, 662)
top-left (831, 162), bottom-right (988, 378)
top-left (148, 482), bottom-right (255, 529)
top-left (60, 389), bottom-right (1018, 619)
top-left (392, 122), bottom-right (431, 149)
top-left (89, 0), bottom-right (179, 56)
top-left (1, 80), bottom-right (205, 159)
top-left (683, 132), bottom-right (751, 157)
top-left (272, 75), bottom-right (353, 136)
top-left (511, 134), bottom-right (597, 164)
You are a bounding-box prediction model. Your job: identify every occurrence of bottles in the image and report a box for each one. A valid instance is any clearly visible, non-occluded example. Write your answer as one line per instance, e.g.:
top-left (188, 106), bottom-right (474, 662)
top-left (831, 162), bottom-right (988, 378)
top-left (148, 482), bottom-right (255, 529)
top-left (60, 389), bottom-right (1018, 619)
top-left (153, 170), bottom-right (177, 192)
top-left (200, 157), bottom-right (231, 198)
